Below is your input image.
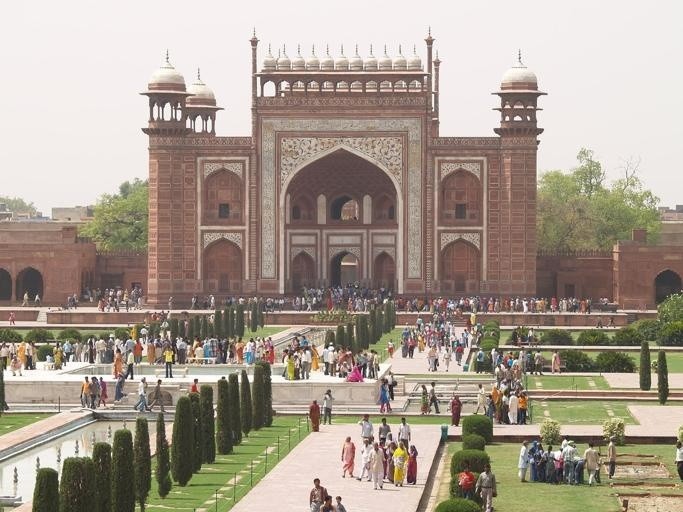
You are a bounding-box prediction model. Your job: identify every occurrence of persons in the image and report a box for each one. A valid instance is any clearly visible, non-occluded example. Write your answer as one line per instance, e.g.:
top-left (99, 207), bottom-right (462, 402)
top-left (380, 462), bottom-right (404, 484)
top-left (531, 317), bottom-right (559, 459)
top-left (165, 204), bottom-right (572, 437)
top-left (309, 400), bottom-right (320, 432)
top-left (458, 464), bottom-right (497, 512)
top-left (1, 283), bottom-right (617, 426)
top-left (607, 436), bottom-right (616, 479)
top-left (309, 479), bottom-right (346, 512)
top-left (342, 414), bottom-right (418, 490)
top-left (517, 435), bottom-right (602, 486)
top-left (675, 441), bottom-right (683, 481)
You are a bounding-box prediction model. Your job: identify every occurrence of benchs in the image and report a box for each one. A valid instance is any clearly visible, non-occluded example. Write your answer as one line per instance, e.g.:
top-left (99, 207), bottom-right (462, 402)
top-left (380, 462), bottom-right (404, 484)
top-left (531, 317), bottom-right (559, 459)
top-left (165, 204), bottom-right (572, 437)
top-left (154, 368), bottom-right (190, 377)
top-left (43, 362), bottom-right (57, 370)
top-left (184, 357), bottom-right (218, 367)
